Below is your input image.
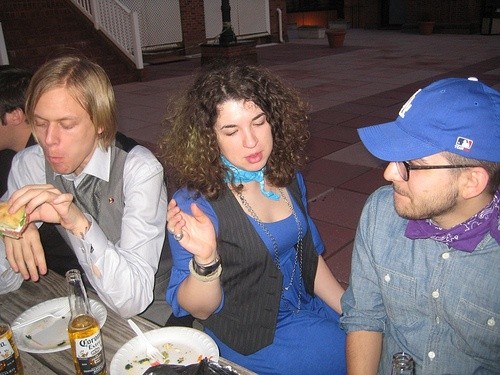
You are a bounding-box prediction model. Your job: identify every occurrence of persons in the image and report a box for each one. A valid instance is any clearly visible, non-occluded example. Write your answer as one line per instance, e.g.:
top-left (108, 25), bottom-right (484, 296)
top-left (0, 55), bottom-right (170, 326)
top-left (340, 77), bottom-right (499, 375)
top-left (163, 61), bottom-right (348, 375)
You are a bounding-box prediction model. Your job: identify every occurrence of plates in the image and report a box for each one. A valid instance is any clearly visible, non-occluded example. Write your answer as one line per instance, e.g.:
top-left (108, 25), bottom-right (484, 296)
top-left (11, 295), bottom-right (107, 354)
top-left (110, 325), bottom-right (220, 375)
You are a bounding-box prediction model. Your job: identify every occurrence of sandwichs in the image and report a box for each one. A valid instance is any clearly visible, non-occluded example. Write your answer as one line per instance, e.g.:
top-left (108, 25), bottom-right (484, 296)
top-left (0, 200), bottom-right (30, 239)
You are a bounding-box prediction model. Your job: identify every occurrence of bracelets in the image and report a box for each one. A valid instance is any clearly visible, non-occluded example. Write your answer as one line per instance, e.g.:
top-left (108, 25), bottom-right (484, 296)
top-left (188, 255), bottom-right (222, 281)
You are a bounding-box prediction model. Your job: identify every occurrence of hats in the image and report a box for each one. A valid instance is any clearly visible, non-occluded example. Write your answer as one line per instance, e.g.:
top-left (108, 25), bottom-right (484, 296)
top-left (356, 75), bottom-right (500, 168)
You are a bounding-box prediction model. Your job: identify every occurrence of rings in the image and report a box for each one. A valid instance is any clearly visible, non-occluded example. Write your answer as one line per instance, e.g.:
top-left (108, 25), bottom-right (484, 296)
top-left (170, 229), bottom-right (182, 242)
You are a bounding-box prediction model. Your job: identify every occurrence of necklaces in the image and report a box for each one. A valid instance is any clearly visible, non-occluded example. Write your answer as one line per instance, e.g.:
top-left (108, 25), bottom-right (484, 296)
top-left (225, 177), bottom-right (302, 314)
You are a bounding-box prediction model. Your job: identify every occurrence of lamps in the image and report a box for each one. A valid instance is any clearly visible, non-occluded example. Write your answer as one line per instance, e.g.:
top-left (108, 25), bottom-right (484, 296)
top-left (480, 15), bottom-right (500, 35)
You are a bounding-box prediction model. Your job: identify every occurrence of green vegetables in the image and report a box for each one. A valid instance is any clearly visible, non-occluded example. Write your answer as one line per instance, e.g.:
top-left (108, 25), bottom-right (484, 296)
top-left (125, 352), bottom-right (184, 370)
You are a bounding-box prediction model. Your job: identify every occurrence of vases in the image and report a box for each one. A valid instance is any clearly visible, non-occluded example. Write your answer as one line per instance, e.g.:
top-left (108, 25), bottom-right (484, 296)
top-left (326, 30), bottom-right (347, 48)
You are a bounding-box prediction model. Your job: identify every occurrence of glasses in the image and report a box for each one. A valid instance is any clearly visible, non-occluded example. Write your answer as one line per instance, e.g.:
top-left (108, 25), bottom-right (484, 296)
top-left (395, 159), bottom-right (483, 182)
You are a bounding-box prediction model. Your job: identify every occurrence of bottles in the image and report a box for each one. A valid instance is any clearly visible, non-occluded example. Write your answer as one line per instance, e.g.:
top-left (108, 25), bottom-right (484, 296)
top-left (66, 269), bottom-right (108, 375)
top-left (0, 305), bottom-right (24, 375)
top-left (391, 352), bottom-right (414, 375)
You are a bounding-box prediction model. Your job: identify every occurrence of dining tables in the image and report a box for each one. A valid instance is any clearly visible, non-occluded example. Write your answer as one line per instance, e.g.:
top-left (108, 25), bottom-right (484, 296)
top-left (0, 268), bottom-right (258, 374)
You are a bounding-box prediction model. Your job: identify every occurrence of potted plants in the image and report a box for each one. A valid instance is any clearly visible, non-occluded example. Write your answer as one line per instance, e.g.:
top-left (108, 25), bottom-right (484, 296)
top-left (286, 7), bottom-right (338, 28)
top-left (297, 25), bottom-right (325, 39)
top-left (415, 7), bottom-right (436, 35)
top-left (328, 18), bottom-right (346, 30)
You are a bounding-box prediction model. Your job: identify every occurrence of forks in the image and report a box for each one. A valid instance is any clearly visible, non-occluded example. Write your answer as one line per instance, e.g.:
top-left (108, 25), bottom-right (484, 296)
top-left (11, 304), bottom-right (72, 330)
top-left (127, 319), bottom-right (166, 362)
